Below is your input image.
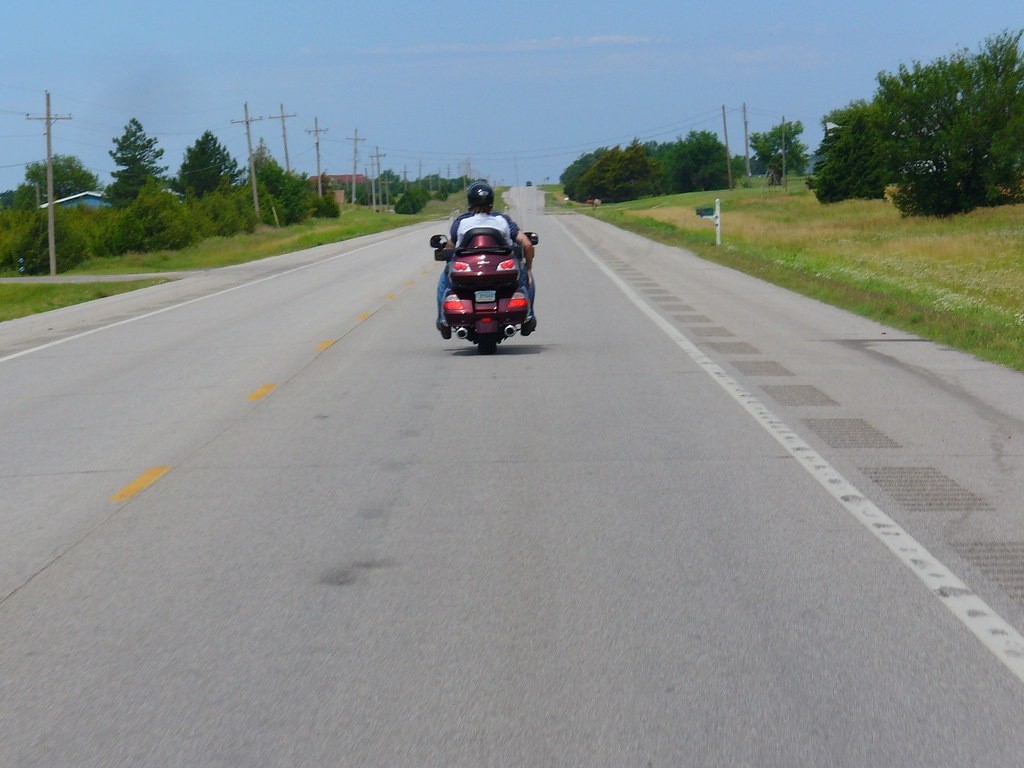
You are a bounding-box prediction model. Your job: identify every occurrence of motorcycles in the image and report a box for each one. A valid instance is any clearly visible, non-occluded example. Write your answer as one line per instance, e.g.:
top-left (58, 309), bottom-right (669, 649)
top-left (429, 227), bottom-right (539, 356)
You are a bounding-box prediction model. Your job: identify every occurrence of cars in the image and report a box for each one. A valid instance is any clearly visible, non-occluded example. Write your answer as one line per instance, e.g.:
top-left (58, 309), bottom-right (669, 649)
top-left (526, 181), bottom-right (532, 186)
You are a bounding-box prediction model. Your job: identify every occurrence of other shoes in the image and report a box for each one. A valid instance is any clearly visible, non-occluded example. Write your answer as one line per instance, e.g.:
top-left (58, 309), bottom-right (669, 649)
top-left (437, 322), bottom-right (451, 339)
top-left (522, 318), bottom-right (536, 336)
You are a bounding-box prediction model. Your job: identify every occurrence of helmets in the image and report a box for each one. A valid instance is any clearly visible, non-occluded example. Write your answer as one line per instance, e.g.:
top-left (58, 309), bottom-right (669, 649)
top-left (467, 181), bottom-right (493, 207)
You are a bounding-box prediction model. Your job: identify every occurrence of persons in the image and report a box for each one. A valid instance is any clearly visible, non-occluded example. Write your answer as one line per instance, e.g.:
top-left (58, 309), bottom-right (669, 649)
top-left (436, 181), bottom-right (537, 339)
